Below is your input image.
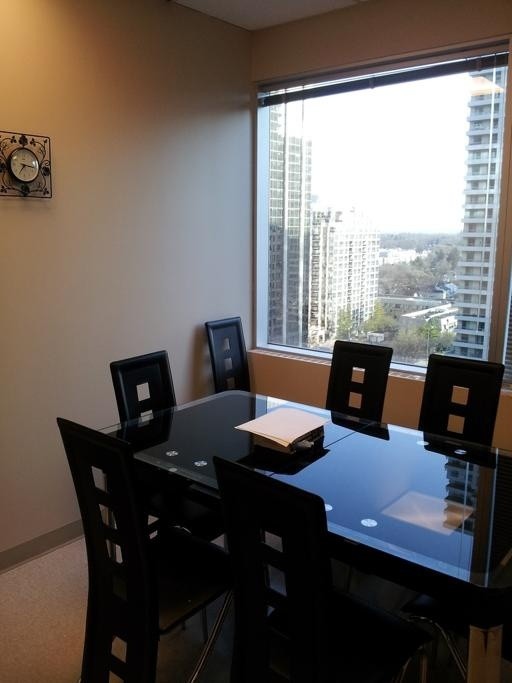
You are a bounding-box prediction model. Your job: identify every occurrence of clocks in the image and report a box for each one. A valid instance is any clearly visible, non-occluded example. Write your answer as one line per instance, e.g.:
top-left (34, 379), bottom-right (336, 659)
top-left (1, 130), bottom-right (53, 199)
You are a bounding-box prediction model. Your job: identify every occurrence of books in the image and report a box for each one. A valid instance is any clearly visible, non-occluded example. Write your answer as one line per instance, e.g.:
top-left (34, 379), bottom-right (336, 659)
top-left (250, 426), bottom-right (325, 456)
